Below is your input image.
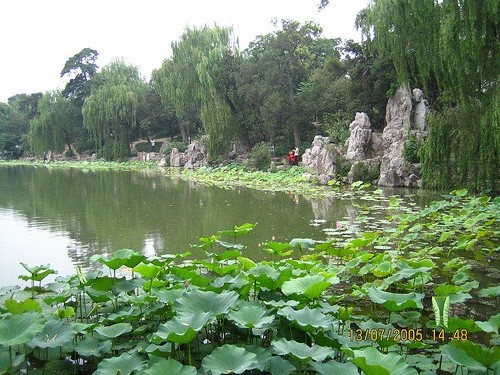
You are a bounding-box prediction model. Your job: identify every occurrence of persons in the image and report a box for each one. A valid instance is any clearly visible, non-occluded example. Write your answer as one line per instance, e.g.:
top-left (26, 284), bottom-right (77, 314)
top-left (293, 146), bottom-right (299, 166)
top-left (288, 148), bottom-right (296, 166)
